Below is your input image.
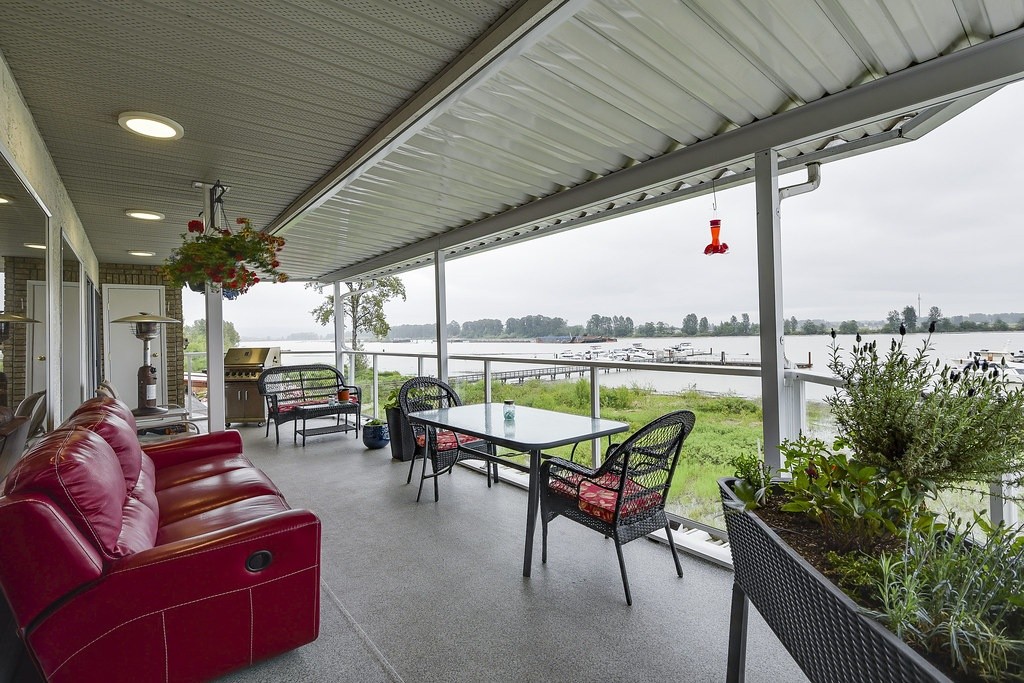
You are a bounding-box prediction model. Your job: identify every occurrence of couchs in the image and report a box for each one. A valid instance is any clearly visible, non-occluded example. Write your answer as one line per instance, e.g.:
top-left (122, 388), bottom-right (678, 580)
top-left (0, 397), bottom-right (321, 683)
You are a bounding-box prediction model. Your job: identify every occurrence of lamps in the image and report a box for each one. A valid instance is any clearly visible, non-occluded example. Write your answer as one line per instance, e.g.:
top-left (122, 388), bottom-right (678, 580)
top-left (107, 311), bottom-right (182, 418)
top-left (0, 311), bottom-right (43, 428)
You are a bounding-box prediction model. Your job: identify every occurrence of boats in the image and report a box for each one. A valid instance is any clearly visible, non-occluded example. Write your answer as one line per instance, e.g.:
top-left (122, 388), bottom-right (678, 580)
top-left (946, 349), bottom-right (1024, 385)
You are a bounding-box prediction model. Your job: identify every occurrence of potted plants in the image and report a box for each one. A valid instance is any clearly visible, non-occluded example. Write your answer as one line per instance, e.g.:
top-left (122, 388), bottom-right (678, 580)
top-left (363, 419), bottom-right (391, 450)
top-left (382, 386), bottom-right (435, 462)
top-left (720, 318), bottom-right (1024, 683)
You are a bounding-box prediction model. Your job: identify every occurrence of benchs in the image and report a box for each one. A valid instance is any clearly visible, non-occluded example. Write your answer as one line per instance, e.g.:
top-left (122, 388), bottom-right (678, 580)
top-left (257, 365), bottom-right (362, 445)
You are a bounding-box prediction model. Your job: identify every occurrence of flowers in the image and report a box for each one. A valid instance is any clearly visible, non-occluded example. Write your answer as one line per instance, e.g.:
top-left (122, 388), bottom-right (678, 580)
top-left (164, 217), bottom-right (294, 290)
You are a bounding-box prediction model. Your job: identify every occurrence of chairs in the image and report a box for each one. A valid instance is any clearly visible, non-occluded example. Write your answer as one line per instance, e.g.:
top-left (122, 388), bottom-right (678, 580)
top-left (539, 410), bottom-right (696, 606)
top-left (0, 389), bottom-right (47, 485)
top-left (398, 378), bottom-right (493, 506)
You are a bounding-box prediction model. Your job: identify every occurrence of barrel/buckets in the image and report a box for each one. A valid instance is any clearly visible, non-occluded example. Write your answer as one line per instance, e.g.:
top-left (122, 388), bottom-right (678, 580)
top-left (338, 389), bottom-right (349, 403)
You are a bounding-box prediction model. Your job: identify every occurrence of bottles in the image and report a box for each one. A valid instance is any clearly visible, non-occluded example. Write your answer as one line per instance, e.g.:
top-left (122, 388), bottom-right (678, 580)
top-left (503, 400), bottom-right (515, 420)
top-left (329, 395), bottom-right (335, 406)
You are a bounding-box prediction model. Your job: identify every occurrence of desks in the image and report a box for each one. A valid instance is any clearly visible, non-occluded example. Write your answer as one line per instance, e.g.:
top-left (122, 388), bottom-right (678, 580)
top-left (130, 408), bottom-right (189, 436)
top-left (407, 402), bottom-right (630, 577)
top-left (294, 402), bottom-right (359, 448)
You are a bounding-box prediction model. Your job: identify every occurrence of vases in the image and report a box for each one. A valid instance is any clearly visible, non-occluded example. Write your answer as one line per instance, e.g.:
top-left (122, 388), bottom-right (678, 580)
top-left (187, 278), bottom-right (209, 292)
